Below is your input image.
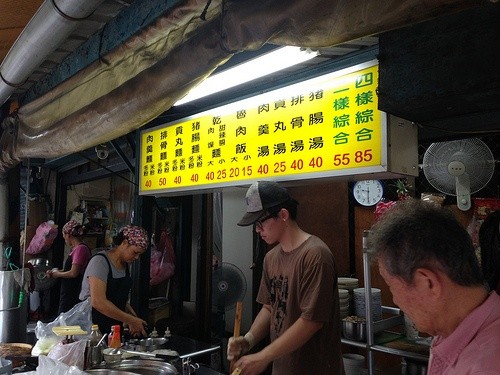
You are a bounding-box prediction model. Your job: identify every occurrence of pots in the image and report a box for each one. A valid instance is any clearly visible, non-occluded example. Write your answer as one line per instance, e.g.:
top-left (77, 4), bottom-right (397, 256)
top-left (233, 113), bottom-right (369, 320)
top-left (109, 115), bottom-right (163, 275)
top-left (82, 359), bottom-right (178, 375)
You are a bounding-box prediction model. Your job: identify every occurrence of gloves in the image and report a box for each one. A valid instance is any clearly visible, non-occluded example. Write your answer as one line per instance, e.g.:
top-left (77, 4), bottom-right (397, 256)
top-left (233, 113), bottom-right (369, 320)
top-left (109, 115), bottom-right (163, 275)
top-left (228, 332), bottom-right (255, 360)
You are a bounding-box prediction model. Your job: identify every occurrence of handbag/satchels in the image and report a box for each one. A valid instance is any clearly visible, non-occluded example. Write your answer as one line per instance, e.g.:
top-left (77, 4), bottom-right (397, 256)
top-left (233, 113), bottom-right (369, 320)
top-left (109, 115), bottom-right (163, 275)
top-left (149, 233), bottom-right (175, 286)
top-left (26, 224), bottom-right (59, 255)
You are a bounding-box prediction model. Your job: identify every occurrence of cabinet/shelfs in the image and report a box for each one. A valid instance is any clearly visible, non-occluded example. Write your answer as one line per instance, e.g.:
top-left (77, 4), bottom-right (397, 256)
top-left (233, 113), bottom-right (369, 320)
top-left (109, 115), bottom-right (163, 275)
top-left (81, 198), bottom-right (109, 235)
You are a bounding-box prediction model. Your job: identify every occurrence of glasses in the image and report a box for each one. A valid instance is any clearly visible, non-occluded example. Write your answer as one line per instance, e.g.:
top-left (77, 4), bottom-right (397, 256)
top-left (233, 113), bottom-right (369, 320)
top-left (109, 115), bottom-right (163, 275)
top-left (255, 207), bottom-right (286, 228)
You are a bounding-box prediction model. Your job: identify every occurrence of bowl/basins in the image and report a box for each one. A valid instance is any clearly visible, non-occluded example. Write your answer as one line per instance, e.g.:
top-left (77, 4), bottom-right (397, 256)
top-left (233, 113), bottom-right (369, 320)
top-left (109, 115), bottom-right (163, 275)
top-left (0, 359), bottom-right (12, 375)
top-left (342, 318), bottom-right (367, 341)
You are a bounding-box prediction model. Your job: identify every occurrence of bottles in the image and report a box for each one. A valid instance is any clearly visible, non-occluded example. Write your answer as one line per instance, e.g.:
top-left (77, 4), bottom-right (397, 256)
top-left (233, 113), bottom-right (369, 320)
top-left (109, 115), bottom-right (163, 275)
top-left (108, 325), bottom-right (173, 356)
top-left (86, 325), bottom-right (105, 370)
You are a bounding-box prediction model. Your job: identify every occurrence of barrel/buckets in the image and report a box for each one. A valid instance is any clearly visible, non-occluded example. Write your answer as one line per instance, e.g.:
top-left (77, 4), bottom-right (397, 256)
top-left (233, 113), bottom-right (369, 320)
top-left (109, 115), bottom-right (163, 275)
top-left (343, 354), bottom-right (366, 375)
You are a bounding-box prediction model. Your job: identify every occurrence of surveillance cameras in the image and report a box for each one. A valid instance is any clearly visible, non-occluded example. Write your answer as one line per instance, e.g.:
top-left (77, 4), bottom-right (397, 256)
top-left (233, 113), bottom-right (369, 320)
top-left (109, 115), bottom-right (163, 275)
top-left (95, 143), bottom-right (109, 160)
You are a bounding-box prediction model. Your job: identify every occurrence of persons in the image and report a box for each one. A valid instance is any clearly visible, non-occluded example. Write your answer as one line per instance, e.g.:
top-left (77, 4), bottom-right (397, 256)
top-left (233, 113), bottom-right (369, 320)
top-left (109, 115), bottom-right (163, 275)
top-left (363, 198), bottom-right (500, 374)
top-left (75, 224), bottom-right (149, 347)
top-left (223, 179), bottom-right (347, 375)
top-left (43, 221), bottom-right (93, 324)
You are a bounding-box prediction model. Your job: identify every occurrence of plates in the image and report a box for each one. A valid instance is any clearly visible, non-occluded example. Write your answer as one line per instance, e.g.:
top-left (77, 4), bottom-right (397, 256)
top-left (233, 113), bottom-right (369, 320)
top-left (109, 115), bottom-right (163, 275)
top-left (336, 277), bottom-right (381, 322)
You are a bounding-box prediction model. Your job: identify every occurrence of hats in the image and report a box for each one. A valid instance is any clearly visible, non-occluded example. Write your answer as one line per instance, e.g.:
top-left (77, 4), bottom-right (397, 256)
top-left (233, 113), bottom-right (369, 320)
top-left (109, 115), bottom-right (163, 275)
top-left (237, 181), bottom-right (290, 226)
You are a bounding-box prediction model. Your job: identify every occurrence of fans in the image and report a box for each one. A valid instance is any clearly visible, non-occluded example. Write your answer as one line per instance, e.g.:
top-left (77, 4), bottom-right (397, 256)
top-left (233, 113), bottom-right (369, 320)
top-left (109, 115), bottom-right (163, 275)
top-left (423, 138), bottom-right (494, 211)
top-left (210, 262), bottom-right (247, 354)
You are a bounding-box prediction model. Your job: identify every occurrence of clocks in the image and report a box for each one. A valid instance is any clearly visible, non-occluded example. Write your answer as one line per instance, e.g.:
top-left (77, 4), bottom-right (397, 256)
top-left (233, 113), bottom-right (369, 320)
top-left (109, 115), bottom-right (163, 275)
top-left (351, 179), bottom-right (385, 209)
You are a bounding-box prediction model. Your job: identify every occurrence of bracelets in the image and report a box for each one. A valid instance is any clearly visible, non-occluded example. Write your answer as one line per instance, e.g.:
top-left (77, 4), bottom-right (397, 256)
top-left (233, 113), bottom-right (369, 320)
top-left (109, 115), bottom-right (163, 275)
top-left (243, 333), bottom-right (254, 349)
